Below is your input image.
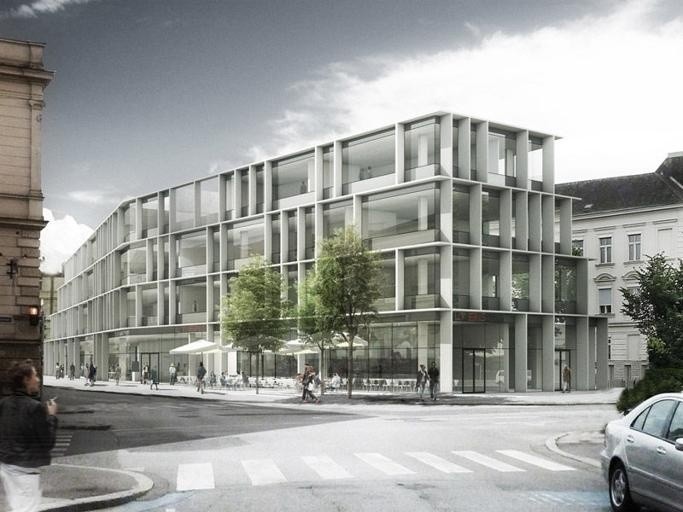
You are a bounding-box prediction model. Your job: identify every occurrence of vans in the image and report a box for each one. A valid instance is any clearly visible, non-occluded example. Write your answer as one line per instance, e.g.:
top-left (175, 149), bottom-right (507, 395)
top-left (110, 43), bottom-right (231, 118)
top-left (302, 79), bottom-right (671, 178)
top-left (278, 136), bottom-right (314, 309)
top-left (496, 369), bottom-right (532, 388)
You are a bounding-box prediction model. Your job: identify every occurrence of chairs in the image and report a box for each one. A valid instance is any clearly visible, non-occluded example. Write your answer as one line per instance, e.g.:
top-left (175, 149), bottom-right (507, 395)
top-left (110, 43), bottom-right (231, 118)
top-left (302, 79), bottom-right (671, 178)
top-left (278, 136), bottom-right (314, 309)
top-left (177, 375), bottom-right (417, 391)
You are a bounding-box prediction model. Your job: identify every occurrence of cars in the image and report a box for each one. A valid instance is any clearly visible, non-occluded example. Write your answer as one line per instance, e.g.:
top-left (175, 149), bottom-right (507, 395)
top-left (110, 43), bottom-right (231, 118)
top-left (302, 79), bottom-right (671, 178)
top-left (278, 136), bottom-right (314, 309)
top-left (600, 393), bottom-right (683, 512)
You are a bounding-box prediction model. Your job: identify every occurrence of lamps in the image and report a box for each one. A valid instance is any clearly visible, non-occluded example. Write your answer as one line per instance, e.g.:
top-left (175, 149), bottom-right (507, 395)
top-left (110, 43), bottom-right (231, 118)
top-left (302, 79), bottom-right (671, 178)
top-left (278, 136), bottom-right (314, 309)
top-left (29, 305), bottom-right (39, 325)
